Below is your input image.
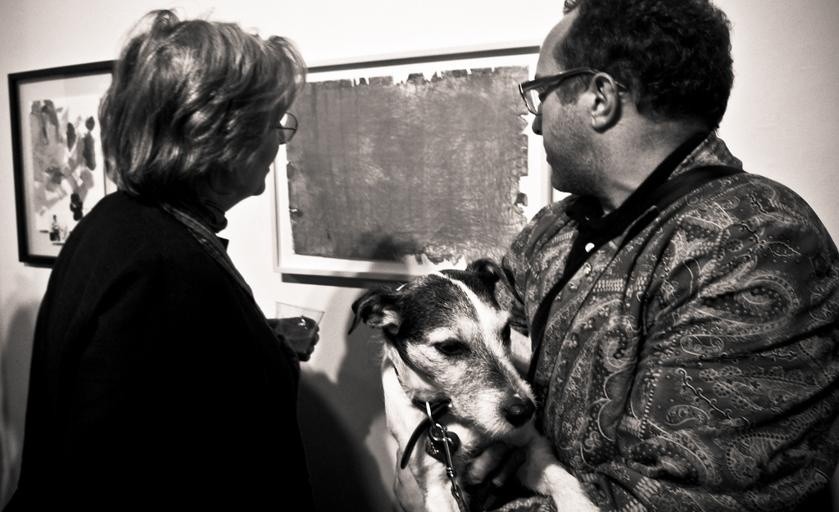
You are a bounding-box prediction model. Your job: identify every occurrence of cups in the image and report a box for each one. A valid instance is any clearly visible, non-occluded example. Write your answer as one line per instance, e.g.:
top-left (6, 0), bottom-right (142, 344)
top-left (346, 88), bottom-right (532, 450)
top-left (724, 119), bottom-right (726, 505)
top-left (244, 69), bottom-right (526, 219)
top-left (272, 302), bottom-right (325, 363)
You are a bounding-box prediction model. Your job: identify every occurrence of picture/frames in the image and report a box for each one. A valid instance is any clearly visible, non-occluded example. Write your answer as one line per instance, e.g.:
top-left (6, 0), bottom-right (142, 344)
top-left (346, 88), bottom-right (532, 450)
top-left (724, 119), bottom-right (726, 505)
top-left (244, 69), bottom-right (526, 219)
top-left (7, 61), bottom-right (119, 266)
top-left (274, 45), bottom-right (554, 288)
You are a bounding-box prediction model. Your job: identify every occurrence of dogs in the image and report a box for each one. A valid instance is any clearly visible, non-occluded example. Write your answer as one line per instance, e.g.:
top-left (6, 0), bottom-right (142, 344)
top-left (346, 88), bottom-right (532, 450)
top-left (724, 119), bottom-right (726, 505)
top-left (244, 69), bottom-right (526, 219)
top-left (347, 257), bottom-right (601, 512)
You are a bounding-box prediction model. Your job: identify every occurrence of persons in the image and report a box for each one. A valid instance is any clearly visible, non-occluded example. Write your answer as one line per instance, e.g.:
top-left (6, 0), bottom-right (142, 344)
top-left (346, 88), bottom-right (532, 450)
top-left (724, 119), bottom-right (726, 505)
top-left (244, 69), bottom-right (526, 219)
top-left (463, 2), bottom-right (839, 512)
top-left (0, 7), bottom-right (322, 512)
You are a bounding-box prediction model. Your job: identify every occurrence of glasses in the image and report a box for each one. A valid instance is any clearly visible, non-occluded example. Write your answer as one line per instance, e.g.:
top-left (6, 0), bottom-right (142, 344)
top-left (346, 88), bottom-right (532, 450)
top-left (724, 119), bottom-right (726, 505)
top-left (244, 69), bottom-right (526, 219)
top-left (519, 67), bottom-right (627, 116)
top-left (267, 112), bottom-right (298, 144)
